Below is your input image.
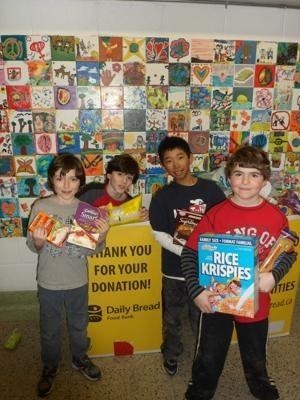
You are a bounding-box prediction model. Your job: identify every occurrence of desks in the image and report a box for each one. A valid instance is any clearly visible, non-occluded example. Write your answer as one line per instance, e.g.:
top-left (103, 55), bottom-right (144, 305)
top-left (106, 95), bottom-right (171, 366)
top-left (87, 222), bottom-right (163, 358)
top-left (231, 214), bottom-right (300, 342)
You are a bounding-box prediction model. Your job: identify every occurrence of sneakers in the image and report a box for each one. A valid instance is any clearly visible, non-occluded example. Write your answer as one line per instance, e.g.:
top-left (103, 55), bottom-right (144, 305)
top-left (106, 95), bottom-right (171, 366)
top-left (162, 357), bottom-right (178, 376)
top-left (71, 355), bottom-right (101, 381)
top-left (37, 366), bottom-right (59, 397)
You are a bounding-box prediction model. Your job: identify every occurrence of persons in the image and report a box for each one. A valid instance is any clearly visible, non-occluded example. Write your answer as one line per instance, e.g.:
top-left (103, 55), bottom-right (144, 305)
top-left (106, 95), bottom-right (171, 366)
top-left (178, 146), bottom-right (297, 400)
top-left (73, 154), bottom-right (151, 225)
top-left (26, 152), bottom-right (111, 398)
top-left (148, 136), bottom-right (228, 376)
